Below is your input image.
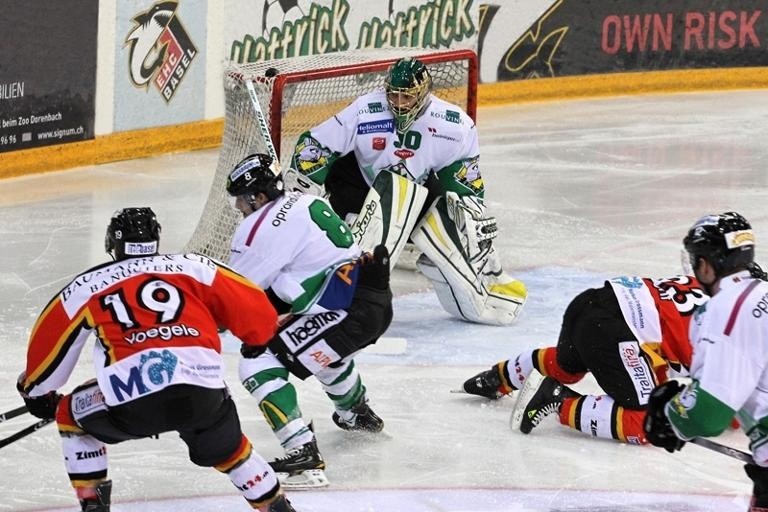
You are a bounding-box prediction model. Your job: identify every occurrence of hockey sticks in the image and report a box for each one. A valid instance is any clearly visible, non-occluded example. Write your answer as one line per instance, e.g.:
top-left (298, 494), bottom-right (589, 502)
top-left (150, 433), bottom-right (321, 473)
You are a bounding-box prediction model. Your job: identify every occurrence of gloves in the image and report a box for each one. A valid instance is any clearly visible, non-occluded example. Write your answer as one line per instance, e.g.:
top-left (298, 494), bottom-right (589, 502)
top-left (237, 340), bottom-right (269, 360)
top-left (642, 378), bottom-right (693, 454)
top-left (15, 370), bottom-right (62, 420)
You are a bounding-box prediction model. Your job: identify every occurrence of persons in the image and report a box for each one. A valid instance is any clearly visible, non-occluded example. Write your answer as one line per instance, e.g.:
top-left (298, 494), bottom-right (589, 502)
top-left (224, 151), bottom-right (393, 475)
top-left (463, 273), bottom-right (710, 445)
top-left (283, 58), bottom-right (529, 325)
top-left (17, 206), bottom-right (297, 511)
top-left (643, 214), bottom-right (768, 512)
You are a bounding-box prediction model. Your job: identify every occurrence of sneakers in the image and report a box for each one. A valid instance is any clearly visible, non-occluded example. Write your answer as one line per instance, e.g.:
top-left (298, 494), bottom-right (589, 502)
top-left (266, 494), bottom-right (296, 510)
top-left (76, 479), bottom-right (113, 512)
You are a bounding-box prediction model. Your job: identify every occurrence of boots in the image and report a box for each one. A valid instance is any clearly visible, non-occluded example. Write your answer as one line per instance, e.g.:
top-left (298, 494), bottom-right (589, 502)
top-left (260, 420), bottom-right (326, 475)
top-left (332, 407), bottom-right (385, 434)
top-left (461, 366), bottom-right (508, 401)
top-left (519, 371), bottom-right (584, 434)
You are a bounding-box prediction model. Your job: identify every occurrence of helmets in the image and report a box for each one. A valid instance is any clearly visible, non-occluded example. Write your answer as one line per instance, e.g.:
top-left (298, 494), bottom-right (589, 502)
top-left (683, 210), bottom-right (756, 279)
top-left (381, 59), bottom-right (433, 123)
top-left (226, 152), bottom-right (284, 200)
top-left (103, 206), bottom-right (161, 259)
